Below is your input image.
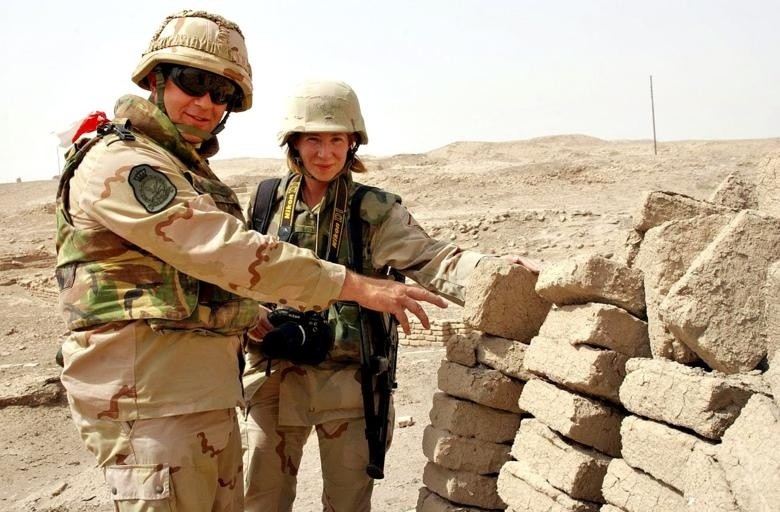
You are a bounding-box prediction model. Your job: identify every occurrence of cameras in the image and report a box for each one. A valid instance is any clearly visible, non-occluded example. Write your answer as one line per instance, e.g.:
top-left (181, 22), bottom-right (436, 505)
top-left (261, 311), bottom-right (333, 366)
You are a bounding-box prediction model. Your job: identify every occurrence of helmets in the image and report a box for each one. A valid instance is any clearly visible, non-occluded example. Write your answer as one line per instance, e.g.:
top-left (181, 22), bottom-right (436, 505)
top-left (276, 82), bottom-right (369, 148)
top-left (132, 9), bottom-right (252, 112)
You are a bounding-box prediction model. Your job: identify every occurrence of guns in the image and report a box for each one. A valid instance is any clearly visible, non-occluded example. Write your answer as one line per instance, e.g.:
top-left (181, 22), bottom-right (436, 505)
top-left (357, 264), bottom-right (406, 479)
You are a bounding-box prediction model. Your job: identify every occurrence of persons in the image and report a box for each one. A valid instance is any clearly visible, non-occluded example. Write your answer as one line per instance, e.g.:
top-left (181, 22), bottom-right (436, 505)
top-left (50, 12), bottom-right (449, 512)
top-left (231, 76), bottom-right (541, 512)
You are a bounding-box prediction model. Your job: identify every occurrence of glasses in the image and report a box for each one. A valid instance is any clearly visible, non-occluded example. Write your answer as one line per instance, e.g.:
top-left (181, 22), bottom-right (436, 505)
top-left (163, 62), bottom-right (238, 105)
